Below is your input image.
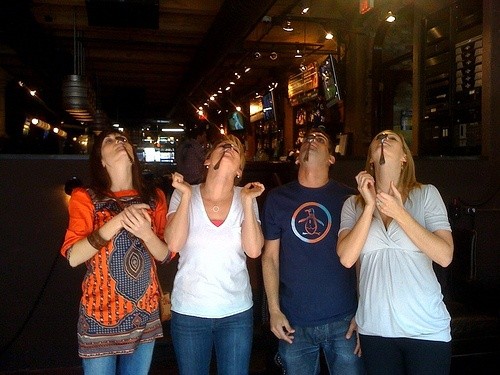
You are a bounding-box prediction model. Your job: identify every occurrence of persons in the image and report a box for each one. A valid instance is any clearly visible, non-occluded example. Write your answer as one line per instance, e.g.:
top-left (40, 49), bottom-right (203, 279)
top-left (162, 135), bottom-right (264, 375)
top-left (180, 127), bottom-right (207, 185)
top-left (60, 129), bottom-right (177, 375)
top-left (336, 129), bottom-right (454, 375)
top-left (262, 128), bottom-right (361, 375)
top-left (233, 113), bottom-right (243, 130)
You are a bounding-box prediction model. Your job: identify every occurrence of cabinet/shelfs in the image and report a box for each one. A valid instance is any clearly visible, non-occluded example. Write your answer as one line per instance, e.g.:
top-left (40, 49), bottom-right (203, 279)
top-left (292, 99), bottom-right (325, 149)
top-left (254, 117), bottom-right (283, 155)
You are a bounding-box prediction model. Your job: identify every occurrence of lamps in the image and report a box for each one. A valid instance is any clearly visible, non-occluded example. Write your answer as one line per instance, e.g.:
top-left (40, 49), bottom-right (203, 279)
top-left (62, 7), bottom-right (109, 137)
top-left (295, 43), bottom-right (303, 58)
top-left (283, 20), bottom-right (294, 31)
top-left (384, 10), bottom-right (396, 23)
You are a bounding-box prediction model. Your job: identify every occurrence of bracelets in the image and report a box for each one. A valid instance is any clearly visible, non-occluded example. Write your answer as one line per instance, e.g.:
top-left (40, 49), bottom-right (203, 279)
top-left (87, 228), bottom-right (111, 251)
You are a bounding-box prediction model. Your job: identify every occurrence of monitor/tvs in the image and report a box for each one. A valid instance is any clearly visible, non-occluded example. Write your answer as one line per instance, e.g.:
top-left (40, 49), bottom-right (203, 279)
top-left (318, 54), bottom-right (344, 107)
top-left (227, 109), bottom-right (246, 133)
top-left (260, 90), bottom-right (277, 123)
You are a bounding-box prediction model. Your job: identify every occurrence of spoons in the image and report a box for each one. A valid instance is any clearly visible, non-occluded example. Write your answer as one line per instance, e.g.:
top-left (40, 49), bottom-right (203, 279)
top-left (304, 135), bottom-right (316, 162)
top-left (377, 134), bottom-right (388, 165)
top-left (214, 144), bottom-right (232, 170)
top-left (115, 136), bottom-right (133, 163)
